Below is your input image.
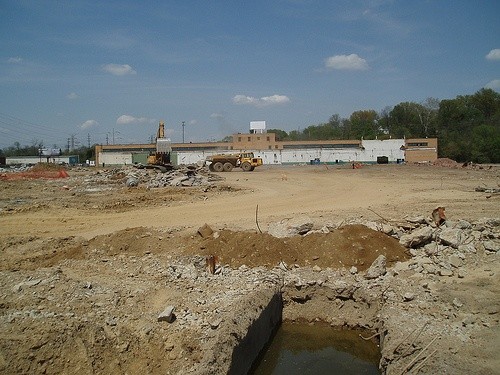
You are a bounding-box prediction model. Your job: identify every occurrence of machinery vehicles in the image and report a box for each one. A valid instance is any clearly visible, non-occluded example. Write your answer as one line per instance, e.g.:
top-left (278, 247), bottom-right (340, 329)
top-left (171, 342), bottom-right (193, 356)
top-left (146, 121), bottom-right (173, 167)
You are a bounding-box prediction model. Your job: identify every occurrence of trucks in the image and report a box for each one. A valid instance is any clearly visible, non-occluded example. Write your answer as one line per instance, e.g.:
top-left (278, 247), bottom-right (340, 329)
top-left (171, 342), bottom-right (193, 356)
top-left (205, 151), bottom-right (262, 172)
